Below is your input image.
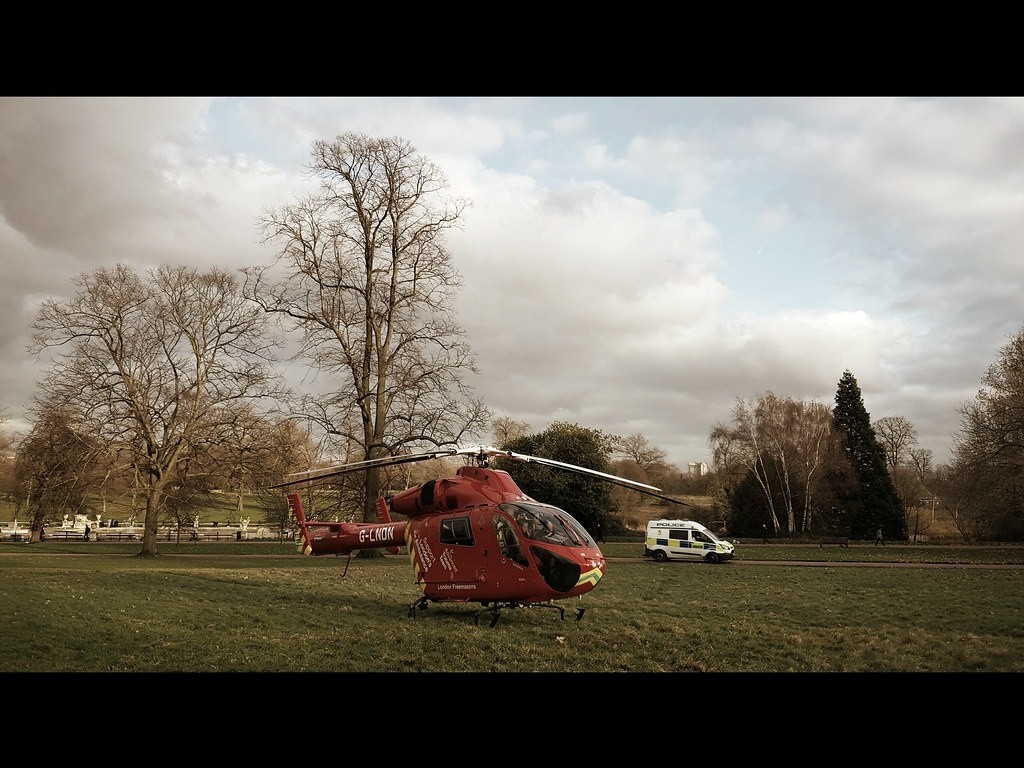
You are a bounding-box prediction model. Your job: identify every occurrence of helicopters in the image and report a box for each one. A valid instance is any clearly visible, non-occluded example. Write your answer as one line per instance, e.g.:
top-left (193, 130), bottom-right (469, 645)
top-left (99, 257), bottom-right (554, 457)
top-left (268, 444), bottom-right (693, 628)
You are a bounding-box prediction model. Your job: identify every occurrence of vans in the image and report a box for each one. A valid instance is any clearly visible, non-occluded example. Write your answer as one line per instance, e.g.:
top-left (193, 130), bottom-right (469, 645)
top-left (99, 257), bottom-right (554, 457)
top-left (642, 518), bottom-right (736, 564)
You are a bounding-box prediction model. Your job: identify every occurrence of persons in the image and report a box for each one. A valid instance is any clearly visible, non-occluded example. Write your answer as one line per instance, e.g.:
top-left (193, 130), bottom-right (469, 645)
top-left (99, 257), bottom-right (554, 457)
top-left (594, 523), bottom-right (605, 544)
top-left (543, 521), bottom-right (569, 546)
top-left (875, 527), bottom-right (886, 546)
top-left (513, 518), bottom-right (541, 567)
top-left (692, 533), bottom-right (697, 541)
top-left (84, 525), bottom-right (90, 541)
top-left (762, 523), bottom-right (770, 544)
